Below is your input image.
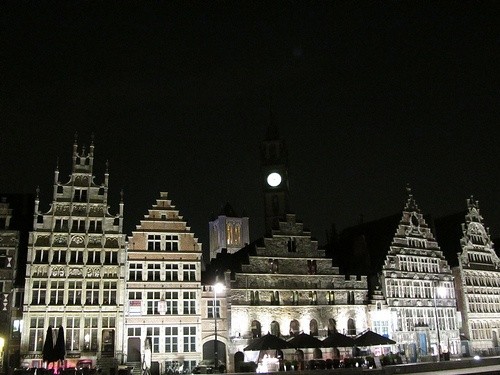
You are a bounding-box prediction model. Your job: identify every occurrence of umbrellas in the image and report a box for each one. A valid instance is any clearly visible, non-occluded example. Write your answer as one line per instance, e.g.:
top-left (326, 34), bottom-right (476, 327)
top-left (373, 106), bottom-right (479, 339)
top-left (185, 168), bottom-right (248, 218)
top-left (355, 330), bottom-right (397, 346)
top-left (287, 333), bottom-right (325, 349)
top-left (243, 331), bottom-right (294, 350)
top-left (320, 333), bottom-right (356, 347)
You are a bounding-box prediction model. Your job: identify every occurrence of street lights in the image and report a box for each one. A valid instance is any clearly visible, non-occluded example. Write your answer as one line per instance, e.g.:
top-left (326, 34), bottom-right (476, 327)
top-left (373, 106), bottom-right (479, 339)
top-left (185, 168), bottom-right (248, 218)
top-left (211, 282), bottom-right (227, 372)
top-left (431, 274), bottom-right (446, 361)
